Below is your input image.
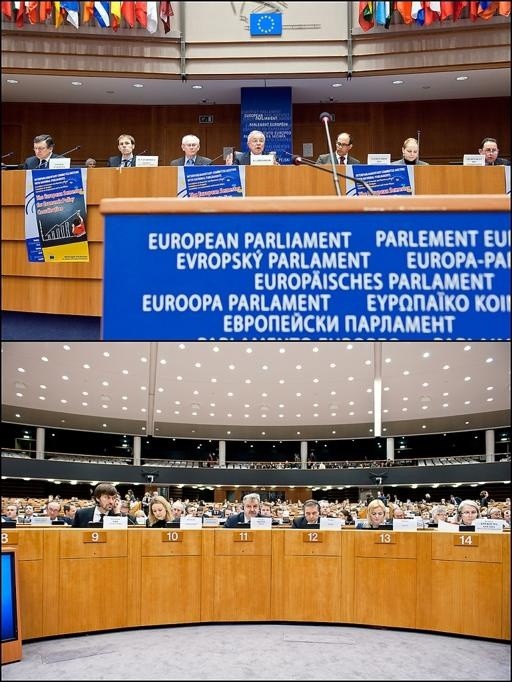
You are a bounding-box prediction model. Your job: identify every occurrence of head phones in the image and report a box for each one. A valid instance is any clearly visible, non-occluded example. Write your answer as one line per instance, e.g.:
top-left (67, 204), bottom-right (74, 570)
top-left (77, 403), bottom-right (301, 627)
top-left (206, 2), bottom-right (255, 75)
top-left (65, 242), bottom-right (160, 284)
top-left (301, 499), bottom-right (322, 515)
top-left (240, 504), bottom-right (244, 510)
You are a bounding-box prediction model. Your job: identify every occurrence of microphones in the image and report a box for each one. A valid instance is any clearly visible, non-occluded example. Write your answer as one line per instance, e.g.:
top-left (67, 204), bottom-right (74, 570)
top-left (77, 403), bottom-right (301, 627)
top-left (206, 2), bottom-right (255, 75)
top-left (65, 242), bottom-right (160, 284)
top-left (291, 154), bottom-right (301, 166)
top-left (120, 510), bottom-right (135, 525)
top-left (416, 130), bottom-right (421, 164)
top-left (36, 145), bottom-right (81, 169)
top-left (120, 149), bottom-right (148, 167)
top-left (0, 152), bottom-right (14, 161)
top-left (319, 111), bottom-right (333, 126)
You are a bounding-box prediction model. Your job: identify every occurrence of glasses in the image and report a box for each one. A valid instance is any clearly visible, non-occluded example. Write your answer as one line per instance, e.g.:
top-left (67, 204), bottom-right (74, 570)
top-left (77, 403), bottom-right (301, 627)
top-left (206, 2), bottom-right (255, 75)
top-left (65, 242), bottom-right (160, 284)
top-left (337, 142), bottom-right (350, 147)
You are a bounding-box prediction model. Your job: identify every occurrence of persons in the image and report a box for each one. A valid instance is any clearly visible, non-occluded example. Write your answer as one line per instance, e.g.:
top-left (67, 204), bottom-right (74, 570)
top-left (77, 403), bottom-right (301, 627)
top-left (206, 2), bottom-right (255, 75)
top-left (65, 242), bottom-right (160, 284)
top-left (107, 134), bottom-right (136, 167)
top-left (24, 134), bottom-right (65, 169)
top-left (232, 130), bottom-right (280, 165)
top-left (250, 453), bottom-right (395, 469)
top-left (2, 483), bottom-right (512, 529)
top-left (72, 211), bottom-right (87, 241)
top-left (170, 134), bottom-right (213, 166)
top-left (315, 131), bottom-right (360, 164)
top-left (478, 137), bottom-right (512, 165)
top-left (85, 157), bottom-right (96, 168)
top-left (391, 137), bottom-right (430, 165)
top-left (206, 452), bottom-right (218, 469)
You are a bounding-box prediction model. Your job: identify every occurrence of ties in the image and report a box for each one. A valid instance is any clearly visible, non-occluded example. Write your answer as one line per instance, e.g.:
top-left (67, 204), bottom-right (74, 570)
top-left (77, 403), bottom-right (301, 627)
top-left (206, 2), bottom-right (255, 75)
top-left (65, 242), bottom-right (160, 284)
top-left (189, 160), bottom-right (193, 165)
top-left (40, 160), bottom-right (45, 169)
top-left (124, 160), bottom-right (129, 167)
top-left (99, 512), bottom-right (105, 524)
top-left (340, 157), bottom-right (345, 164)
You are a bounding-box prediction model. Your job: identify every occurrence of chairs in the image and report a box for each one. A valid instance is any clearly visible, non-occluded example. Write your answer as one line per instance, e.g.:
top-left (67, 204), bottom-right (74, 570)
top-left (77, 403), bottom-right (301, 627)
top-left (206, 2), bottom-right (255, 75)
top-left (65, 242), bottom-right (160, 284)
top-left (0, 452), bottom-right (248, 470)
top-left (417, 455), bottom-right (482, 467)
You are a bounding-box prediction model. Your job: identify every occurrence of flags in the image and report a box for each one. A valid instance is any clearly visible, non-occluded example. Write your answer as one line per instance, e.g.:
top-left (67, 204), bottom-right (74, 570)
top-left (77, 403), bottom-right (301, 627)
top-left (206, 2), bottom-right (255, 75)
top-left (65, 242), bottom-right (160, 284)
top-left (358, 0), bottom-right (512, 33)
top-left (0, 0), bottom-right (175, 35)
